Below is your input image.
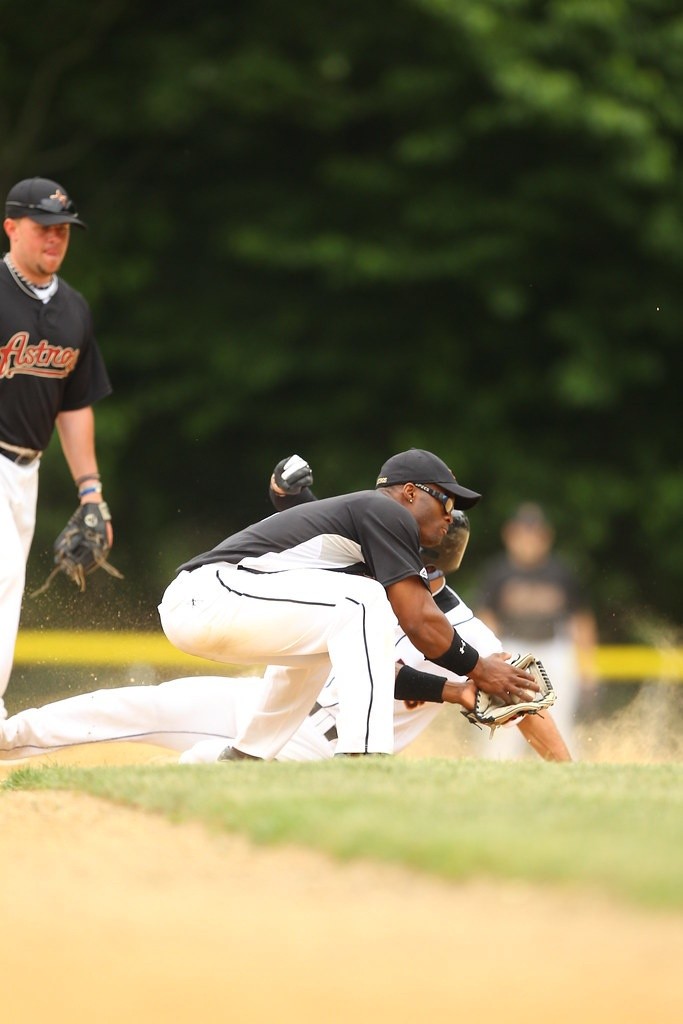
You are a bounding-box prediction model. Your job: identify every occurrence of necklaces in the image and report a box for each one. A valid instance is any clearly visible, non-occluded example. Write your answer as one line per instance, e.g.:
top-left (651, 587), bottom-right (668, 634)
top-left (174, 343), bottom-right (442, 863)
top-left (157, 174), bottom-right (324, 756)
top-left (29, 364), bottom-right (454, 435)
top-left (9, 257), bottom-right (54, 289)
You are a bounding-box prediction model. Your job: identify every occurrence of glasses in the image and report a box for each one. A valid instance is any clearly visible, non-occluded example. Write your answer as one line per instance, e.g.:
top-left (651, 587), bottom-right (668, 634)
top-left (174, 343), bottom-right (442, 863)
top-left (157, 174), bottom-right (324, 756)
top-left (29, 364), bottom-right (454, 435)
top-left (413, 484), bottom-right (455, 515)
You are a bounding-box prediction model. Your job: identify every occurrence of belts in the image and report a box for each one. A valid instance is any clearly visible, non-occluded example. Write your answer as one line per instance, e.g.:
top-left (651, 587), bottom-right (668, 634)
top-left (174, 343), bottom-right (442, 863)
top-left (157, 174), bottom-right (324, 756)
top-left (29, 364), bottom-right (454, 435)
top-left (0, 447), bottom-right (39, 467)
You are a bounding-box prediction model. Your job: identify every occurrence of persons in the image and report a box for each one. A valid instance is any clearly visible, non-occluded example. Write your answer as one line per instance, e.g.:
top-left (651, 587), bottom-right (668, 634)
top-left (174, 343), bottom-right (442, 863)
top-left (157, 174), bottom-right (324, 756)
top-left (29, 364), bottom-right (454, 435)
top-left (0, 176), bottom-right (115, 766)
top-left (0, 448), bottom-right (598, 761)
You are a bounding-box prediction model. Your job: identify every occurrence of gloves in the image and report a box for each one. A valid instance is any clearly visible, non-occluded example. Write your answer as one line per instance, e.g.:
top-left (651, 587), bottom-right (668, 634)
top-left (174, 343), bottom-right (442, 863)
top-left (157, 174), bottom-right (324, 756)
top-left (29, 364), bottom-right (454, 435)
top-left (271, 454), bottom-right (313, 496)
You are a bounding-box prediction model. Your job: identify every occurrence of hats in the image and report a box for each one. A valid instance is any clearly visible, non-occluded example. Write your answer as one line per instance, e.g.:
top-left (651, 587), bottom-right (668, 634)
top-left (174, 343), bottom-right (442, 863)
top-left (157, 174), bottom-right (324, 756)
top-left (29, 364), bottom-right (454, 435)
top-left (375, 447), bottom-right (482, 511)
top-left (5, 176), bottom-right (88, 231)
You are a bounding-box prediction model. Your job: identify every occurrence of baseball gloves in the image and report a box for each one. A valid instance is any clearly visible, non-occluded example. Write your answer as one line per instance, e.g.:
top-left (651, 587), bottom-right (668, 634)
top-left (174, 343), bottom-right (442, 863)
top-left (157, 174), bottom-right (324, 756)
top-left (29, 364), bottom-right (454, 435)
top-left (28, 502), bottom-right (124, 600)
top-left (459, 653), bottom-right (558, 740)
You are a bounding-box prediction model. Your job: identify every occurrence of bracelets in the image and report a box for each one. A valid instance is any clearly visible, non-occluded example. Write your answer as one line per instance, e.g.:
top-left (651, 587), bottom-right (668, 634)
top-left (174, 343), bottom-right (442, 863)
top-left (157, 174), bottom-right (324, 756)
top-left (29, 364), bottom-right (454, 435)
top-left (76, 483), bottom-right (103, 497)
top-left (74, 473), bottom-right (102, 487)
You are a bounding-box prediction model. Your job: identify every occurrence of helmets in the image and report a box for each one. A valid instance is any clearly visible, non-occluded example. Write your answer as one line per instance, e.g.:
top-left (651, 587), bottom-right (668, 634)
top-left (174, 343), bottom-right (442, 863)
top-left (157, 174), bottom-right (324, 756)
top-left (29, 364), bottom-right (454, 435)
top-left (416, 510), bottom-right (471, 583)
